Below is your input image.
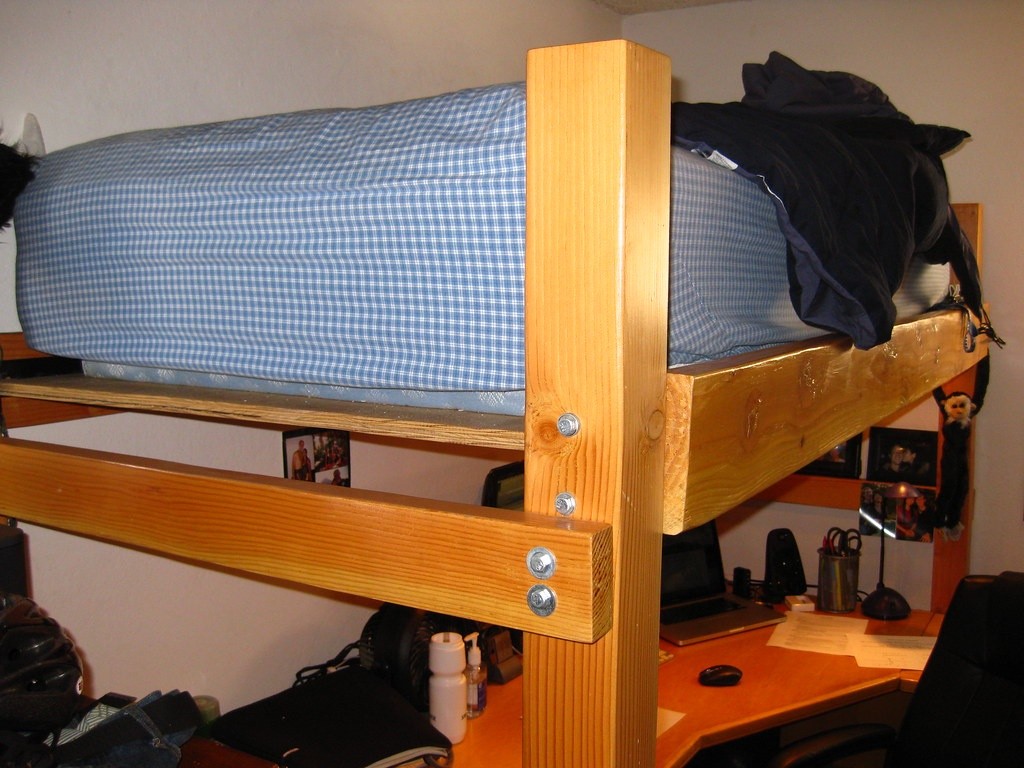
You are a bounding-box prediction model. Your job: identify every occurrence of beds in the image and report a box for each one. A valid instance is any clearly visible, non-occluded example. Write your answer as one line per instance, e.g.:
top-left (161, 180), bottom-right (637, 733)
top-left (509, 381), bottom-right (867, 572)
top-left (0, 39), bottom-right (983, 768)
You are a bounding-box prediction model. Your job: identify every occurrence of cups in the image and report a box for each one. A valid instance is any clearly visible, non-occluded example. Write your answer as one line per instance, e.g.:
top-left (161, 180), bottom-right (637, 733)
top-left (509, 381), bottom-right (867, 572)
top-left (817, 545), bottom-right (862, 614)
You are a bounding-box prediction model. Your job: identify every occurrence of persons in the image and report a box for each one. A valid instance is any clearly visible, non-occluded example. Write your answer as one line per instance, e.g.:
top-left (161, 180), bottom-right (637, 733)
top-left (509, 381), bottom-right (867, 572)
top-left (292, 432), bottom-right (346, 486)
top-left (860, 444), bottom-right (932, 543)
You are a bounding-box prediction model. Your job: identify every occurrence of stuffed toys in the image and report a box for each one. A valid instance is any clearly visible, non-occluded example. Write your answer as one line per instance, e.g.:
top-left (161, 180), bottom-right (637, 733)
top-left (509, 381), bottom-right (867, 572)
top-left (932, 356), bottom-right (990, 533)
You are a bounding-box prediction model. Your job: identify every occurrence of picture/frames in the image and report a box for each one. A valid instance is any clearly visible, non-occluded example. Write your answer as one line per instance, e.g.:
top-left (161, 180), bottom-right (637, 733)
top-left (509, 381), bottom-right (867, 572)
top-left (794, 433), bottom-right (862, 479)
top-left (867, 426), bottom-right (938, 486)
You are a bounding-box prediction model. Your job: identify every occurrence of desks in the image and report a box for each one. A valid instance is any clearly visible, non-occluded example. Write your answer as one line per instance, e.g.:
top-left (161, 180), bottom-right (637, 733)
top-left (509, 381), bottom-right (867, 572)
top-left (438, 594), bottom-right (934, 768)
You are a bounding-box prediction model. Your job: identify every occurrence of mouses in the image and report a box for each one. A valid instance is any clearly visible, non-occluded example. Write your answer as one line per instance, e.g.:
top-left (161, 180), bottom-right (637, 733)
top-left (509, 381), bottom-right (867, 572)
top-left (699, 664), bottom-right (742, 686)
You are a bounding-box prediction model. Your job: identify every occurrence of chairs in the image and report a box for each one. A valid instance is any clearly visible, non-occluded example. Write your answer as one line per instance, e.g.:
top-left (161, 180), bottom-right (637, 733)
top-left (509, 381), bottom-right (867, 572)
top-left (769, 570), bottom-right (1024, 768)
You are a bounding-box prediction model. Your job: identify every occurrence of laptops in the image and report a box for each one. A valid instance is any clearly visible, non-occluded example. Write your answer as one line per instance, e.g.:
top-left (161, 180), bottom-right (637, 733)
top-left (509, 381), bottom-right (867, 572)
top-left (660, 519), bottom-right (788, 647)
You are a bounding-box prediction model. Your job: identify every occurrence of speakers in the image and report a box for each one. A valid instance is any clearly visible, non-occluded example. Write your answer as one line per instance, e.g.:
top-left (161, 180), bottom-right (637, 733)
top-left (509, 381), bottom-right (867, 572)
top-left (764, 528), bottom-right (807, 597)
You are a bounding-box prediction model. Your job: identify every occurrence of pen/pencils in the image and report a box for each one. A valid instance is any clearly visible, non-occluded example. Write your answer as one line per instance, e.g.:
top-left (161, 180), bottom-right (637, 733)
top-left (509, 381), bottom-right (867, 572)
top-left (822, 535), bottom-right (842, 611)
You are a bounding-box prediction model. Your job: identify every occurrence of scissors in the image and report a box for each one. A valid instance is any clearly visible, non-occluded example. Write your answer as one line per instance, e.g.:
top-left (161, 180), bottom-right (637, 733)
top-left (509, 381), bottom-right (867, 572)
top-left (827, 527), bottom-right (861, 604)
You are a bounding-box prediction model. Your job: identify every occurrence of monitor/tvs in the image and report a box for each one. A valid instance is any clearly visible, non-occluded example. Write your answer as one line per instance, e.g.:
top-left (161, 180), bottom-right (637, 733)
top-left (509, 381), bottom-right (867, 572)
top-left (482, 461), bottom-right (525, 514)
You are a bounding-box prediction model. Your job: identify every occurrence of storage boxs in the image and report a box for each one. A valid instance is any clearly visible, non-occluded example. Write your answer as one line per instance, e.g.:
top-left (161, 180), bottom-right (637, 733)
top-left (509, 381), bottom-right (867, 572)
top-left (785, 596), bottom-right (815, 612)
